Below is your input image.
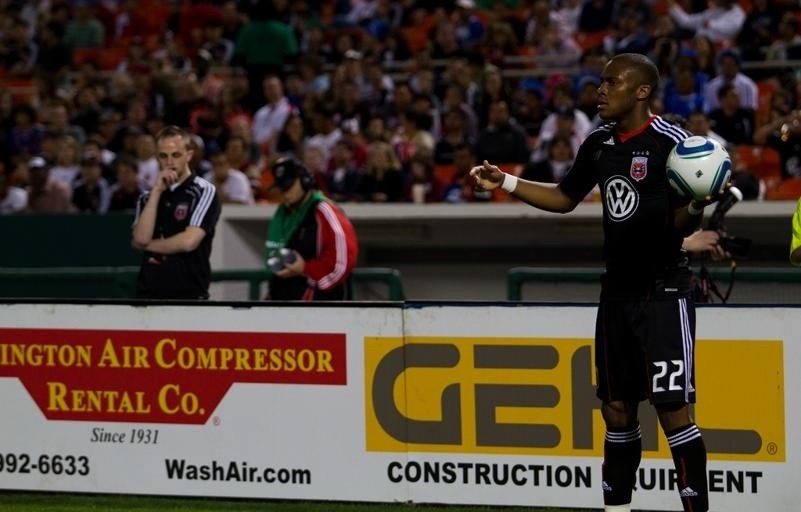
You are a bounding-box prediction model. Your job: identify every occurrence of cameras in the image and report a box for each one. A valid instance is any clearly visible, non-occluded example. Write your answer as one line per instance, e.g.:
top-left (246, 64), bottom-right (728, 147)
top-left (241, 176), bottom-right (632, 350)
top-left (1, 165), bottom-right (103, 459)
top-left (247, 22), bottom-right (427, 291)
top-left (266, 246), bottom-right (295, 273)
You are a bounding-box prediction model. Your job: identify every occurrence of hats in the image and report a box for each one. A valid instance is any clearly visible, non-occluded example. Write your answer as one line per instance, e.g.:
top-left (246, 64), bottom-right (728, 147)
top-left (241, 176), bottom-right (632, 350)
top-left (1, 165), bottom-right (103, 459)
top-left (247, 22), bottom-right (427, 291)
top-left (267, 156), bottom-right (298, 192)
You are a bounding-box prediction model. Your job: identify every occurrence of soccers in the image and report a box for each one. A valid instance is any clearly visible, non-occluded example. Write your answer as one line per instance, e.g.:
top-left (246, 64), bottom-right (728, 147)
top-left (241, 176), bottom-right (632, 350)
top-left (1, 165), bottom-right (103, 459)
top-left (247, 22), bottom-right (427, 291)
top-left (666, 136), bottom-right (732, 201)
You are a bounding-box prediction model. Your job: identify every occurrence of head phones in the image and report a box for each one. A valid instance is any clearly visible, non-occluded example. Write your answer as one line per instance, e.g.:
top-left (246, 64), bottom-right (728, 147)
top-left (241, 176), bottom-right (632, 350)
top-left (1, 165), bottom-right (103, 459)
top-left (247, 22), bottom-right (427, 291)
top-left (276, 155), bottom-right (313, 188)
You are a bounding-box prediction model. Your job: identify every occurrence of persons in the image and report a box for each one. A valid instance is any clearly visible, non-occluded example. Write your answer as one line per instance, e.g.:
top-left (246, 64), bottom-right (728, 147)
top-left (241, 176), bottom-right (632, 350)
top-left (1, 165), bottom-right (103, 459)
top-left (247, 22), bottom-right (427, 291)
top-left (1, 0), bottom-right (801, 216)
top-left (261, 155), bottom-right (361, 302)
top-left (680, 232), bottom-right (732, 305)
top-left (789, 194), bottom-right (801, 268)
top-left (470, 53), bottom-right (732, 509)
top-left (131, 125), bottom-right (218, 298)
